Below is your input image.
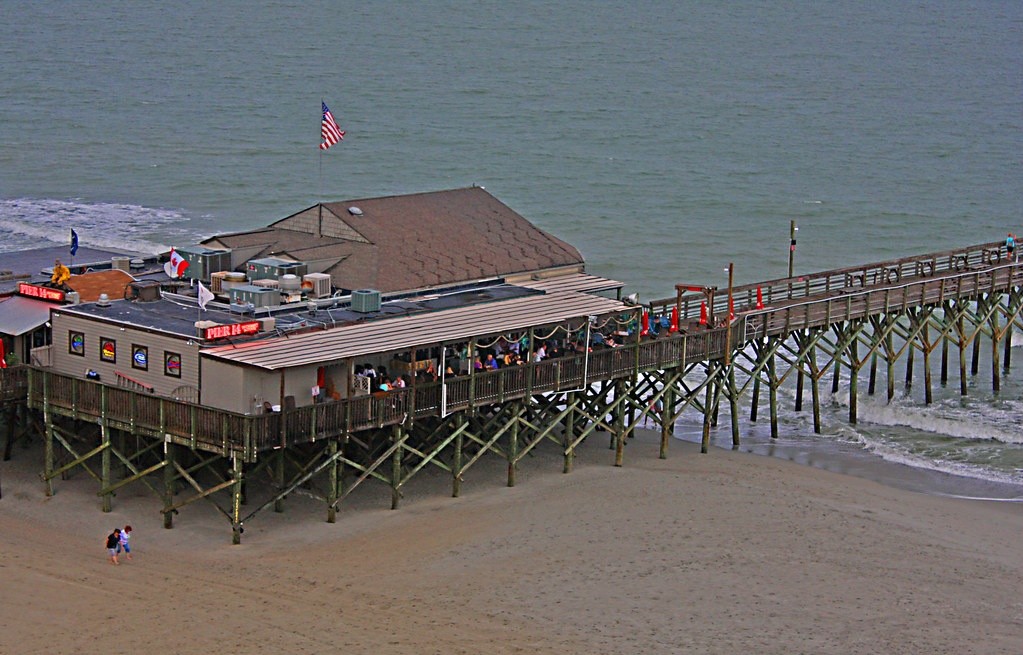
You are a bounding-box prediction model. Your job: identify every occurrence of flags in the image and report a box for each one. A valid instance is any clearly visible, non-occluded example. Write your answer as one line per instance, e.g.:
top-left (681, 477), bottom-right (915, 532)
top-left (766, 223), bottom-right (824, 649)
top-left (198, 281), bottom-right (215, 312)
top-left (170, 248), bottom-right (190, 277)
top-left (320, 101), bottom-right (346, 151)
top-left (70, 228), bottom-right (78, 255)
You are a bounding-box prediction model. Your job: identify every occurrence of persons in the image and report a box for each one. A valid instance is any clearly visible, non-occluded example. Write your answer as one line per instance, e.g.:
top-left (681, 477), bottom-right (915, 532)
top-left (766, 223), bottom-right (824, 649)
top-left (50, 257), bottom-right (71, 289)
top-left (354, 312), bottom-right (659, 408)
top-left (1006, 232), bottom-right (1014, 261)
top-left (104, 529), bottom-right (121, 565)
top-left (115, 526), bottom-right (132, 560)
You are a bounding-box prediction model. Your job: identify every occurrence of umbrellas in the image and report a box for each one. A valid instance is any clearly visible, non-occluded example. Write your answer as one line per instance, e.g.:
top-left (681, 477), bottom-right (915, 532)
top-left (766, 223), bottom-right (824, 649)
top-left (669, 306), bottom-right (678, 331)
top-left (729, 298), bottom-right (734, 320)
top-left (700, 300), bottom-right (706, 324)
top-left (641, 310), bottom-right (649, 336)
top-left (755, 286), bottom-right (764, 310)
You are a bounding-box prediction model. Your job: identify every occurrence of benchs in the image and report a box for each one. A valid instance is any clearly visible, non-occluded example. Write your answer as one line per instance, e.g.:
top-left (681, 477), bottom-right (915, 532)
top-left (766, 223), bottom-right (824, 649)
top-left (966, 263), bottom-right (992, 279)
top-left (834, 286), bottom-right (869, 304)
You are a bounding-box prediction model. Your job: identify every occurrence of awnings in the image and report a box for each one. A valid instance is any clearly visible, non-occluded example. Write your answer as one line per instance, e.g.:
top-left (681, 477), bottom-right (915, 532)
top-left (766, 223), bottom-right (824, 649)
top-left (0, 296), bottom-right (62, 337)
